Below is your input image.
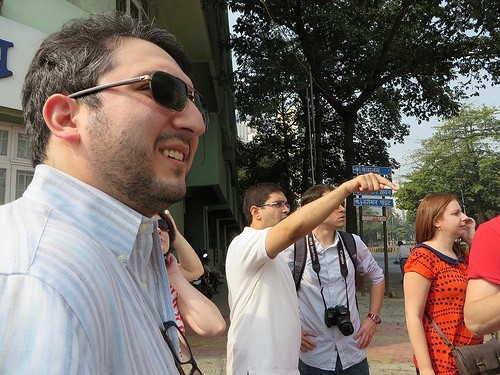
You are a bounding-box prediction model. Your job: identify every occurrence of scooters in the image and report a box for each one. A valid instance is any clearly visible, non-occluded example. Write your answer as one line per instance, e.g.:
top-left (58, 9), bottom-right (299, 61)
top-left (193, 249), bottom-right (220, 298)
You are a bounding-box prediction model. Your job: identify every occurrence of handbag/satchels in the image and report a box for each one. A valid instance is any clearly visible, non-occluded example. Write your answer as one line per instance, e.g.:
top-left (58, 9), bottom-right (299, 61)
top-left (451, 336), bottom-right (500, 375)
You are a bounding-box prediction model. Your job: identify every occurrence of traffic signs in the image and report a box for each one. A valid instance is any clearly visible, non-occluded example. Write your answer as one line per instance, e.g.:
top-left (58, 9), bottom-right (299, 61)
top-left (352, 164), bottom-right (394, 222)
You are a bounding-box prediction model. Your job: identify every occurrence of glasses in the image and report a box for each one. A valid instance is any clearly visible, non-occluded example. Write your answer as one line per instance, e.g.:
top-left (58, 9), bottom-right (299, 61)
top-left (157, 219), bottom-right (170, 232)
top-left (159, 320), bottom-right (203, 375)
top-left (259, 201), bottom-right (291, 208)
top-left (67, 70), bottom-right (208, 131)
top-left (341, 201), bottom-right (345, 207)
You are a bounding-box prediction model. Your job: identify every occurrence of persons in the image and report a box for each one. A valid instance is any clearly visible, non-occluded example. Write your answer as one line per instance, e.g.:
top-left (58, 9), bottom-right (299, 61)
top-left (397, 241), bottom-right (410, 284)
top-left (0, 14), bottom-right (210, 375)
top-left (226, 173), bottom-right (399, 375)
top-left (284, 184), bottom-right (385, 375)
top-left (164, 210), bottom-right (205, 282)
top-left (463, 215), bottom-right (500, 336)
top-left (403, 193), bottom-right (484, 375)
top-left (151, 211), bottom-right (226, 358)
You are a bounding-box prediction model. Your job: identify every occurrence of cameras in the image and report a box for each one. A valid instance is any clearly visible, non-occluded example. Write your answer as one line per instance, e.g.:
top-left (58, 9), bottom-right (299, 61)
top-left (325, 304), bottom-right (354, 336)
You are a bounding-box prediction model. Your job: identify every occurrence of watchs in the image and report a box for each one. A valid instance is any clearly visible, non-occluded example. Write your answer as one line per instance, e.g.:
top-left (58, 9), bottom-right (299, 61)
top-left (367, 313), bottom-right (381, 324)
